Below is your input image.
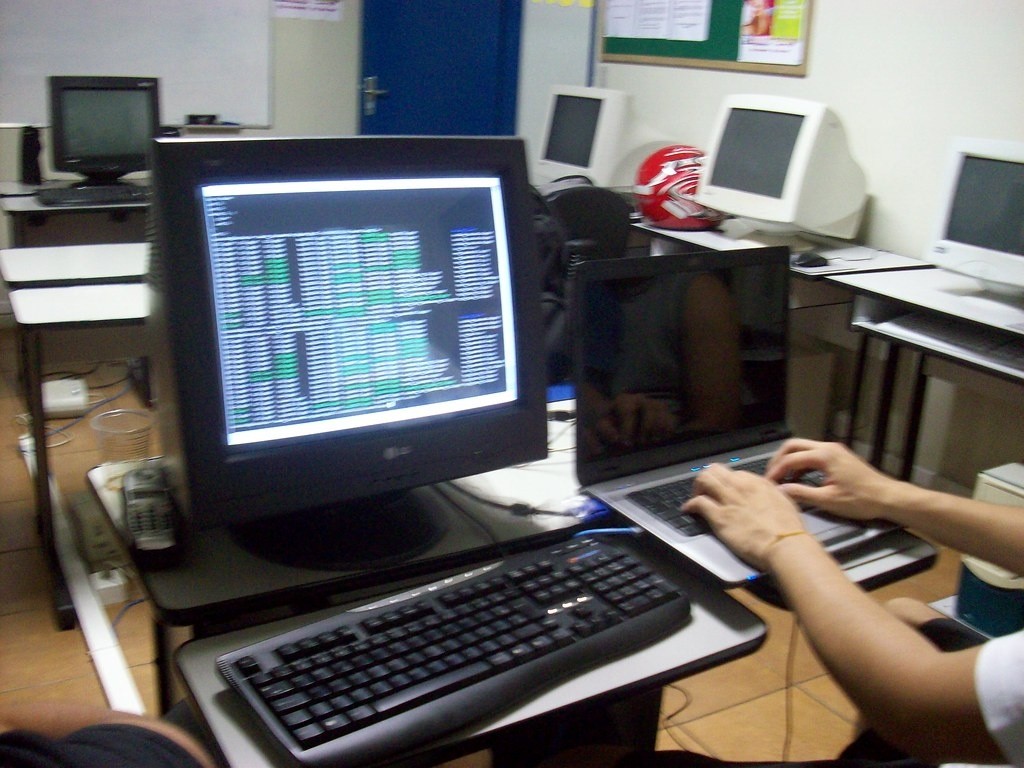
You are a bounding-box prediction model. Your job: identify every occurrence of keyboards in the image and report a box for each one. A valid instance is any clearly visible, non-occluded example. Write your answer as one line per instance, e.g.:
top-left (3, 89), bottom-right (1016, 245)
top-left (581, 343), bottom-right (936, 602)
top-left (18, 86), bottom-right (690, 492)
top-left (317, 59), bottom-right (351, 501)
top-left (217, 536), bottom-right (691, 768)
top-left (37, 185), bottom-right (156, 209)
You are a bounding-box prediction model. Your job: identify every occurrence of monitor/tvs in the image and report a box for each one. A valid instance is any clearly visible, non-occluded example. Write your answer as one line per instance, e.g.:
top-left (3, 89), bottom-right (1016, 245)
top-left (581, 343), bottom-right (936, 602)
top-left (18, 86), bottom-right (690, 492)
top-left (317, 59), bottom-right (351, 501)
top-left (154, 135), bottom-right (548, 572)
top-left (48, 75), bottom-right (160, 187)
top-left (934, 149), bottom-right (1024, 314)
top-left (535, 85), bottom-right (682, 193)
top-left (694, 92), bottom-right (867, 251)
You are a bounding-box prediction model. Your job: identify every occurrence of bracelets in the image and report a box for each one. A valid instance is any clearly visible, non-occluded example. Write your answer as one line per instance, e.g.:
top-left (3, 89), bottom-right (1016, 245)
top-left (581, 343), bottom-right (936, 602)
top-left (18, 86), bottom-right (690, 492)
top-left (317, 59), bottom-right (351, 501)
top-left (762, 530), bottom-right (808, 564)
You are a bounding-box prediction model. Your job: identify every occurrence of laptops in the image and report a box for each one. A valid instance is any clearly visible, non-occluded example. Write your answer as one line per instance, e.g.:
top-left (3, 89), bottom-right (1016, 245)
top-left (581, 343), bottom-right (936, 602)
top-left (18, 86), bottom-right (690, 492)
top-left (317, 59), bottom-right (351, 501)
top-left (570, 247), bottom-right (906, 590)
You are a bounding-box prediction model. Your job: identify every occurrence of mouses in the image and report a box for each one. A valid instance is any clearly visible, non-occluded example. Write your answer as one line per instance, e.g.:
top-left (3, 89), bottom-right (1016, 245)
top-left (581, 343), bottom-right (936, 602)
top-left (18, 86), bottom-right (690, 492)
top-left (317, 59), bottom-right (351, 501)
top-left (792, 250), bottom-right (827, 267)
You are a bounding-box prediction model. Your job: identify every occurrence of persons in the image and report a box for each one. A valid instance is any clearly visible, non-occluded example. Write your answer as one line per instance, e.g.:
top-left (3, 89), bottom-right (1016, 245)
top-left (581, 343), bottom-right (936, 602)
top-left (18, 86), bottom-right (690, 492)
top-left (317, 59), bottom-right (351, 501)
top-left (572, 273), bottom-right (736, 457)
top-left (681, 438), bottom-right (1024, 768)
top-left (0, 700), bottom-right (214, 768)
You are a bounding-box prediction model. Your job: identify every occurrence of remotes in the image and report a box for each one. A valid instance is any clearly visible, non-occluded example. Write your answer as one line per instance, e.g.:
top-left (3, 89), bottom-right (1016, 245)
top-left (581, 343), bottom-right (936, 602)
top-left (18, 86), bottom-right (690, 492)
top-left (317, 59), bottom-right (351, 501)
top-left (123, 466), bottom-right (185, 571)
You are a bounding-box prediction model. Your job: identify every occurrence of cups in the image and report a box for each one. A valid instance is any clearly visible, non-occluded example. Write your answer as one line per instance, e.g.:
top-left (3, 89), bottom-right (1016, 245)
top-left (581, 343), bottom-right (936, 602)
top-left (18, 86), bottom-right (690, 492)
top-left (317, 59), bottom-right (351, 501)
top-left (91, 408), bottom-right (154, 464)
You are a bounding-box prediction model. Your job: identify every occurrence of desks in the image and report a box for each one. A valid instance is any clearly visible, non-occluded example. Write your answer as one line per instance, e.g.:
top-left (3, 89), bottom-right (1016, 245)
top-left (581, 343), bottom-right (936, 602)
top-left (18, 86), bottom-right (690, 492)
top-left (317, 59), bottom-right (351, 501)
top-left (631, 217), bottom-right (936, 473)
top-left (0, 243), bottom-right (153, 632)
top-left (86, 399), bottom-right (940, 768)
top-left (827, 268), bottom-right (1024, 484)
top-left (0, 178), bottom-right (151, 249)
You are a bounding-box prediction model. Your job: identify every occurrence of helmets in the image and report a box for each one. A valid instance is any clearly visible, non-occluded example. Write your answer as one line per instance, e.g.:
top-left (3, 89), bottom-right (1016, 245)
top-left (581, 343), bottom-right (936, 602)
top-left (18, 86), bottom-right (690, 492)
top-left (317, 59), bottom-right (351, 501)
top-left (633, 144), bottom-right (724, 229)
